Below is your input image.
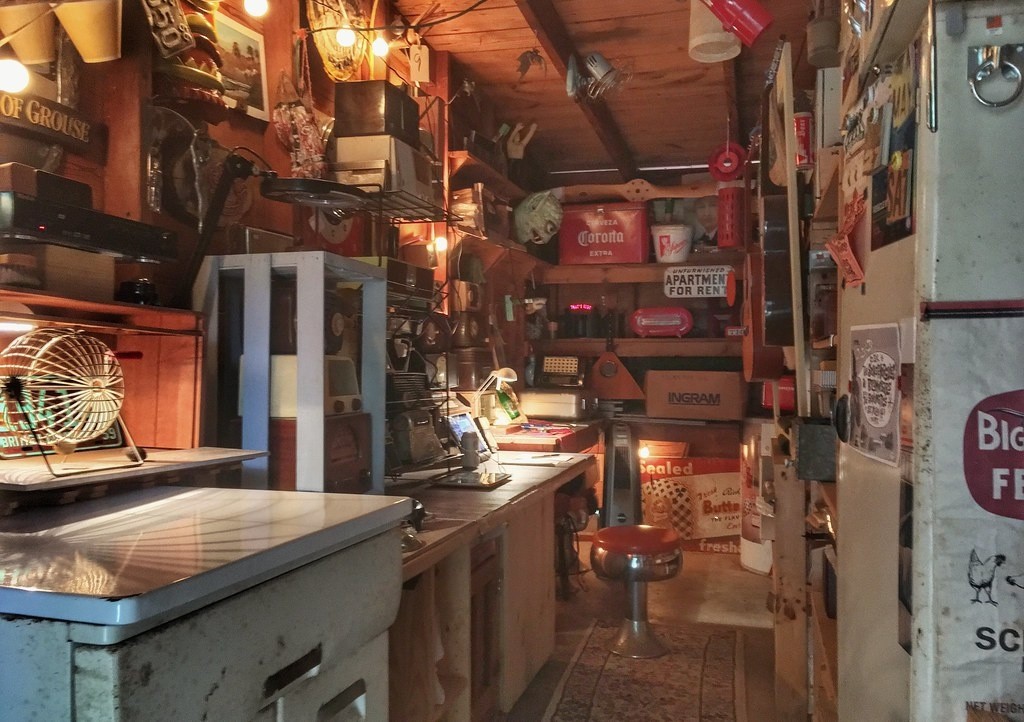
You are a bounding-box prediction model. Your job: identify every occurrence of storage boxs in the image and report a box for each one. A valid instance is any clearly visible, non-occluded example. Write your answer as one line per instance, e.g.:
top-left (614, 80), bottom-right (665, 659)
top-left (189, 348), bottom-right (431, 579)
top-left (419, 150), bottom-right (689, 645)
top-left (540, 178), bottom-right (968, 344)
top-left (641, 370), bottom-right (747, 422)
top-left (556, 203), bottom-right (653, 265)
top-left (641, 456), bottom-right (741, 553)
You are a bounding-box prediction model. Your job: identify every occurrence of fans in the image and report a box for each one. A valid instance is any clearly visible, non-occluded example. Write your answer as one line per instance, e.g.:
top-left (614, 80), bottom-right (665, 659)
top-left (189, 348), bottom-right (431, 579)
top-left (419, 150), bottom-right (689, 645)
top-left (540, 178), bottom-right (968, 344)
top-left (565, 51), bottom-right (638, 102)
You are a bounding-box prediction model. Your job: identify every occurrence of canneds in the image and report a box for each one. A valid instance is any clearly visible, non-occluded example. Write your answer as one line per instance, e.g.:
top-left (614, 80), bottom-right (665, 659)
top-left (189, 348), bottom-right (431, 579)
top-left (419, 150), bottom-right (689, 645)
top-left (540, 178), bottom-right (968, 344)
top-left (793, 111), bottom-right (815, 170)
top-left (461, 432), bottom-right (480, 469)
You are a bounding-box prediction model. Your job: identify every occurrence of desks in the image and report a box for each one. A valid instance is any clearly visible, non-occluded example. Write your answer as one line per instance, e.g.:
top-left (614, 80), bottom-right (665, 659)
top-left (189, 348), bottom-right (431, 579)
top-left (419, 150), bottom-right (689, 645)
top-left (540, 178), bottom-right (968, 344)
top-left (376, 451), bottom-right (598, 721)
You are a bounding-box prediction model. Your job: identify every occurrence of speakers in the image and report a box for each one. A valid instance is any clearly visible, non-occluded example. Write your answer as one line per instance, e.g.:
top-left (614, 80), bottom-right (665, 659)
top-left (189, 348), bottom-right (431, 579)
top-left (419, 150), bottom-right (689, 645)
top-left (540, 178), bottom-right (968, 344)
top-left (391, 410), bottom-right (436, 465)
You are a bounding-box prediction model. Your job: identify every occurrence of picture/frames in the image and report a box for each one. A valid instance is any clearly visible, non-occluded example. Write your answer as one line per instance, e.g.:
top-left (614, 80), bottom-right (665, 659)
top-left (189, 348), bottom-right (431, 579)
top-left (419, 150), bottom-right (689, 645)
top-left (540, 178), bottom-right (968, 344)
top-left (212, 9), bottom-right (269, 125)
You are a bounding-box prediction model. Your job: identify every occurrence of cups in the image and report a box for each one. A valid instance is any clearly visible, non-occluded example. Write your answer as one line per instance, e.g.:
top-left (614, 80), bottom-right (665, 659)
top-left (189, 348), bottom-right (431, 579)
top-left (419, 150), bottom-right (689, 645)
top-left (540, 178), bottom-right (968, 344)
top-left (794, 111), bottom-right (816, 166)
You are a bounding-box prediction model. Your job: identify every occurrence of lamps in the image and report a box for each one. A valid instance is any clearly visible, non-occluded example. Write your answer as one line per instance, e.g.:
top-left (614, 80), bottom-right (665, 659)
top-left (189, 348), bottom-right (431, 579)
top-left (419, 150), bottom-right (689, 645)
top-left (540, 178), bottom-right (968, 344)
top-left (167, 143), bottom-right (386, 308)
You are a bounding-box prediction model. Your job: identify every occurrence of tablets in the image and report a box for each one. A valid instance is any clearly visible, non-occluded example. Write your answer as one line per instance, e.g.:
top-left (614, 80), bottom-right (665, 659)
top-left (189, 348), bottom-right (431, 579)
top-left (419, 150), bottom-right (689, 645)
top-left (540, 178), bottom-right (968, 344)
top-left (443, 412), bottom-right (492, 464)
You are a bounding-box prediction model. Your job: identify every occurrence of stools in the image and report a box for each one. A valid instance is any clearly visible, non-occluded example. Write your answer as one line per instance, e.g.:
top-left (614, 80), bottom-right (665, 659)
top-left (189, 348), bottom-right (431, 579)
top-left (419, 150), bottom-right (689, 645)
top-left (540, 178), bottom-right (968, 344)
top-left (589, 523), bottom-right (685, 658)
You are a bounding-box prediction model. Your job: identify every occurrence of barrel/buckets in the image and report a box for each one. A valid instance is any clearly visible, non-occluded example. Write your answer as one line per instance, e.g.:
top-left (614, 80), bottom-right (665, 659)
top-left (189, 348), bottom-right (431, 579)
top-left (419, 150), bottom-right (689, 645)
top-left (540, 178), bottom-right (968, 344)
top-left (650, 224), bottom-right (693, 264)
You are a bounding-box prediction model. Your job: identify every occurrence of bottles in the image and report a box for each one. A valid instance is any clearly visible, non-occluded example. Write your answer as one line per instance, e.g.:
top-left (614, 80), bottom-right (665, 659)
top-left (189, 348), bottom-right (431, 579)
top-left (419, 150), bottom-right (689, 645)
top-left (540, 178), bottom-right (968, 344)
top-left (497, 387), bottom-right (520, 419)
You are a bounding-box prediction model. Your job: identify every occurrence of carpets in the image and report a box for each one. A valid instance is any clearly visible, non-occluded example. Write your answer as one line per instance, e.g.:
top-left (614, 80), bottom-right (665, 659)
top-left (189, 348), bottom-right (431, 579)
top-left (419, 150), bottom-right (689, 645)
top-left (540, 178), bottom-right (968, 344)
top-left (539, 615), bottom-right (745, 722)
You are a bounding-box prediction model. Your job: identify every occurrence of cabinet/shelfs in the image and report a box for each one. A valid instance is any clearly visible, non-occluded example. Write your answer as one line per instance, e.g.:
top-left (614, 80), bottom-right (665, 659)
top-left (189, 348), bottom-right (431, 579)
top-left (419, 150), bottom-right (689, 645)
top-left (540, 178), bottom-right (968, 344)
top-left (411, 51), bottom-right (552, 395)
top-left (0, 289), bottom-right (274, 505)
top-left (529, 177), bottom-right (758, 425)
